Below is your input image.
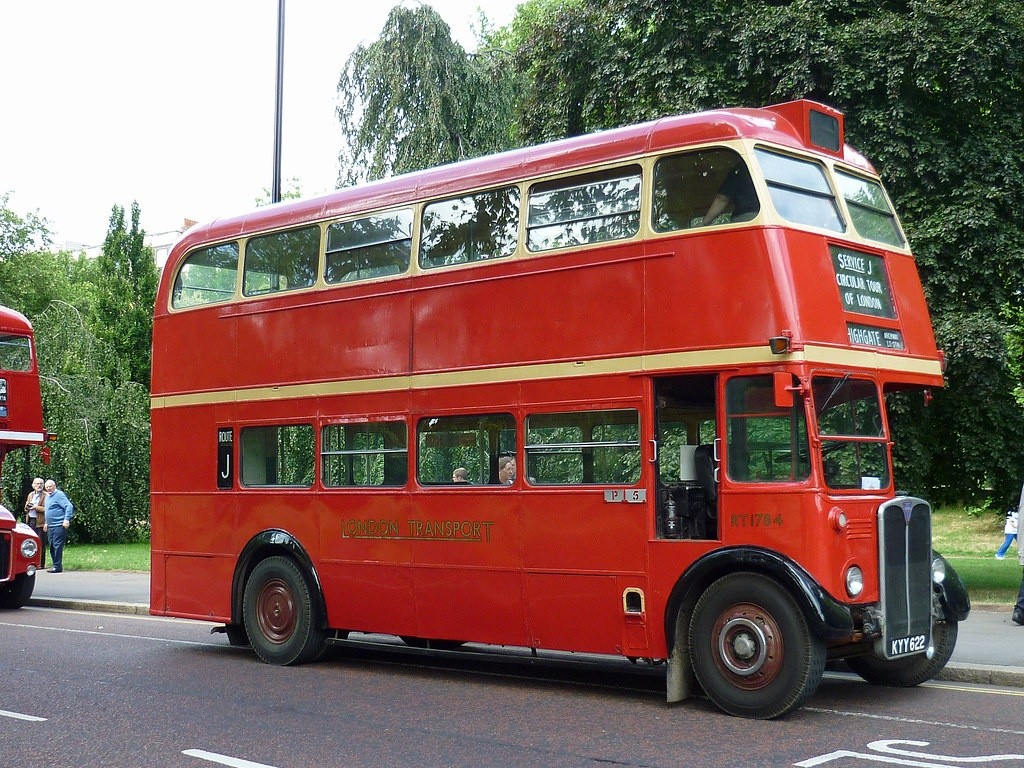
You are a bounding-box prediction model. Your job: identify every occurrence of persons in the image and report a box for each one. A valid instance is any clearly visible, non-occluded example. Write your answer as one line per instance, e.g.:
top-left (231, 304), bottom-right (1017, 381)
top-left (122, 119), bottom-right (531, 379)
top-left (499, 456), bottom-right (516, 484)
top-left (996, 510), bottom-right (1018, 559)
top-left (453, 468), bottom-right (467, 482)
top-left (1012, 484), bottom-right (1024, 625)
top-left (43, 480), bottom-right (73, 572)
top-left (24, 478), bottom-right (47, 570)
top-left (693, 161), bottom-right (760, 228)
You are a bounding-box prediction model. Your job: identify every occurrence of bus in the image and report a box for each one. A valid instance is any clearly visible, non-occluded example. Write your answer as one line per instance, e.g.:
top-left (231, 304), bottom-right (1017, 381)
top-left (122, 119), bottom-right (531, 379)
top-left (0, 306), bottom-right (57, 608)
top-left (148, 98), bottom-right (970, 721)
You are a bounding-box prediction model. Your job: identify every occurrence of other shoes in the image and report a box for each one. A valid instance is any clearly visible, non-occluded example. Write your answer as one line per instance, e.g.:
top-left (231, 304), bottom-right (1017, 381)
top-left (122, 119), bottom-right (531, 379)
top-left (1013, 605), bottom-right (1024, 625)
top-left (36, 566), bottom-right (45, 570)
top-left (46, 568), bottom-right (63, 572)
top-left (995, 554), bottom-right (1004, 560)
